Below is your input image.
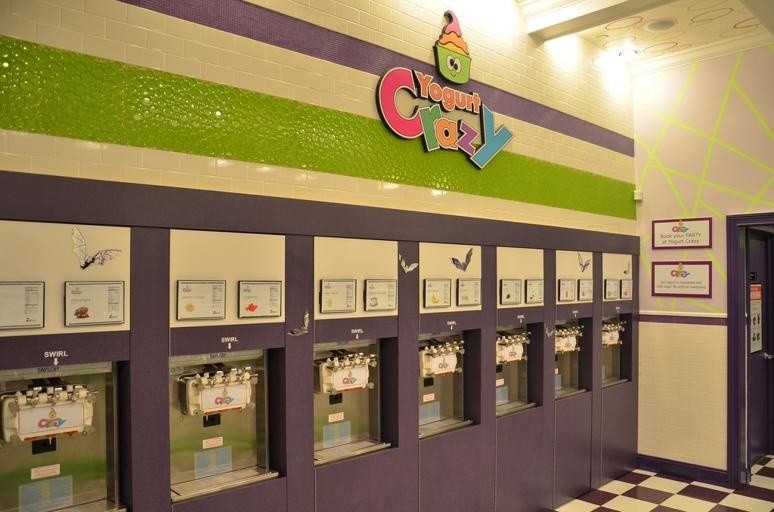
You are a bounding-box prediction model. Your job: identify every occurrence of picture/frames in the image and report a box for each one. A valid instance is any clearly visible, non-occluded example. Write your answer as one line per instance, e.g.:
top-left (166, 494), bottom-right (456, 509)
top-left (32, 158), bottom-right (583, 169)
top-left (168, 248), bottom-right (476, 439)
top-left (651, 216), bottom-right (712, 249)
top-left (651, 260), bottom-right (713, 298)
